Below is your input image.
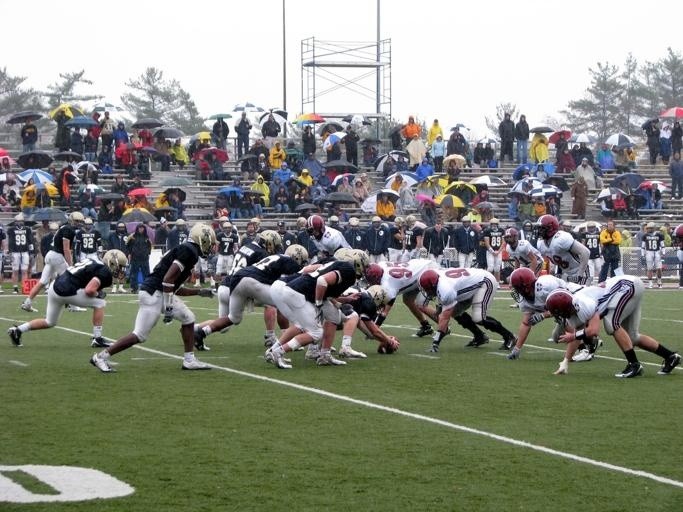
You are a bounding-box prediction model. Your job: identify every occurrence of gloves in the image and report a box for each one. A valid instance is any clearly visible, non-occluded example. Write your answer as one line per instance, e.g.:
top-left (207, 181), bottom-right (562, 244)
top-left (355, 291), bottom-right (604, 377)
top-left (528, 311), bottom-right (544, 325)
top-left (198, 286), bottom-right (216, 299)
top-left (553, 357), bottom-right (570, 376)
top-left (163, 306), bottom-right (175, 322)
top-left (505, 345), bottom-right (520, 360)
top-left (96, 290), bottom-right (107, 300)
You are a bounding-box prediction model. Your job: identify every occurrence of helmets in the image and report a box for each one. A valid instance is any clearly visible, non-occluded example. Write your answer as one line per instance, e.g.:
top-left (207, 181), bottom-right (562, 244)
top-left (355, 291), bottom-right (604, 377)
top-left (187, 223), bottom-right (218, 261)
top-left (503, 228), bottom-right (519, 243)
top-left (545, 291), bottom-right (577, 319)
top-left (68, 211), bottom-right (85, 227)
top-left (489, 218), bottom-right (500, 225)
top-left (579, 222), bottom-right (597, 234)
top-left (221, 221), bottom-right (232, 228)
top-left (15, 214), bottom-right (25, 221)
top-left (218, 216), bottom-right (229, 223)
top-left (249, 217), bottom-right (261, 224)
top-left (256, 229), bottom-right (283, 256)
top-left (306, 215), bottom-right (325, 234)
top-left (329, 214), bottom-right (416, 227)
top-left (175, 218), bottom-right (185, 226)
top-left (417, 269), bottom-right (440, 301)
top-left (102, 249), bottom-right (129, 281)
top-left (509, 267), bottom-right (538, 305)
top-left (333, 247), bottom-right (384, 285)
top-left (532, 214), bottom-right (559, 240)
top-left (671, 223), bottom-right (683, 243)
top-left (462, 215), bottom-right (471, 224)
top-left (297, 217), bottom-right (307, 228)
top-left (284, 244), bottom-right (309, 265)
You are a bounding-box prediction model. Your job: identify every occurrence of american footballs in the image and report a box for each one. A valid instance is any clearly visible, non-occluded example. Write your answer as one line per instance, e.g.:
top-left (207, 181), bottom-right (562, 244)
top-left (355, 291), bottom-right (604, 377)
top-left (378, 336), bottom-right (400, 354)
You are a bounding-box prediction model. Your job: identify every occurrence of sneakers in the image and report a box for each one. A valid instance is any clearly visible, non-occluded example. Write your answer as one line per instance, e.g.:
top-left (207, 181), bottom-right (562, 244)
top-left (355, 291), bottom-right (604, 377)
top-left (180, 324), bottom-right (211, 352)
top-left (90, 352), bottom-right (112, 372)
top-left (112, 287), bottom-right (127, 294)
top-left (658, 353), bottom-right (681, 375)
top-left (7, 325), bottom-right (24, 348)
top-left (12, 287), bottom-right (20, 294)
top-left (68, 304), bottom-right (89, 312)
top-left (499, 336), bottom-right (517, 350)
top-left (90, 337), bottom-right (115, 348)
top-left (181, 357), bottom-right (211, 370)
top-left (463, 334), bottom-right (489, 349)
top-left (264, 322), bottom-right (452, 370)
top-left (572, 336), bottom-right (603, 363)
top-left (613, 363), bottom-right (644, 379)
top-left (21, 302), bottom-right (39, 314)
top-left (647, 282), bottom-right (663, 289)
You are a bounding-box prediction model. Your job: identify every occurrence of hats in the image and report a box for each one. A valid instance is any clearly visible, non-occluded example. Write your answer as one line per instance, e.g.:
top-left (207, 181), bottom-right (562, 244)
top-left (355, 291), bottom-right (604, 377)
top-left (646, 222), bottom-right (655, 228)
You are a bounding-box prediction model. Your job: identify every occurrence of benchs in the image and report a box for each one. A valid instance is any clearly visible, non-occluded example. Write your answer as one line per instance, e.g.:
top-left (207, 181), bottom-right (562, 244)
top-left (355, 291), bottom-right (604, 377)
top-left (0, 132), bottom-right (683, 277)
